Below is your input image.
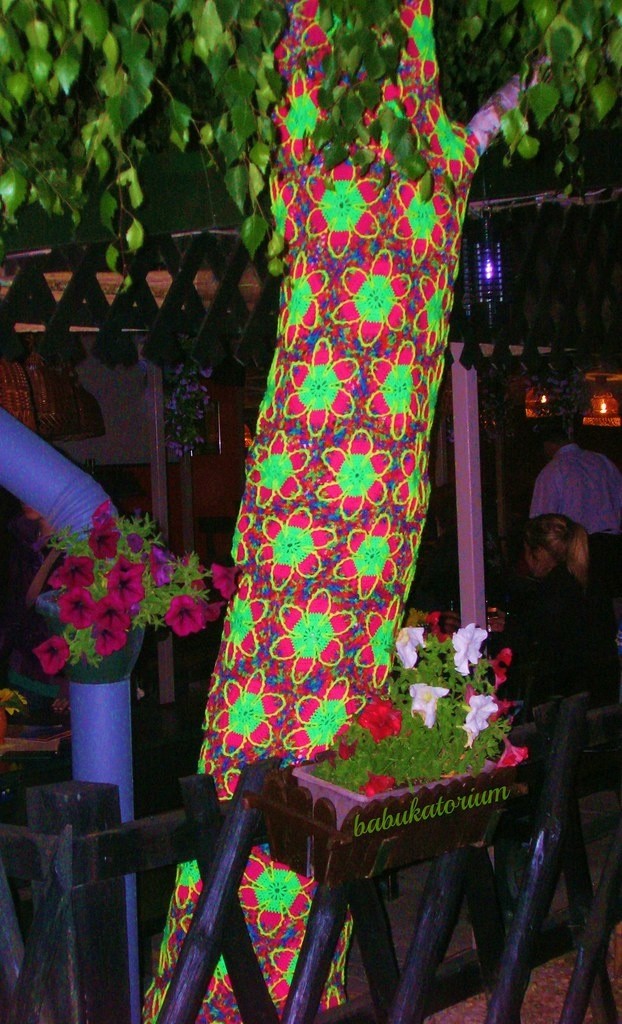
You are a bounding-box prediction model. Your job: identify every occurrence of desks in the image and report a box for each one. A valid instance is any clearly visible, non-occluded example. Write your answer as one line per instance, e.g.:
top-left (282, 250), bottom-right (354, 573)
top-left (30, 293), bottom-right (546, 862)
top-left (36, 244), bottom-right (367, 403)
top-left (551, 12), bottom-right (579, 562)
top-left (0, 724), bottom-right (73, 757)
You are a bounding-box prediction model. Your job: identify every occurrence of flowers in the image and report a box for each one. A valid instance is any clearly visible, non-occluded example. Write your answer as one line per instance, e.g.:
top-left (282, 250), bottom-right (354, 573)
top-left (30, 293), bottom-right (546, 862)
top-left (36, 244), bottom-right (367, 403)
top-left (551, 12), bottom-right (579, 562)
top-left (0, 688), bottom-right (28, 716)
top-left (162, 358), bottom-right (212, 464)
top-left (32, 499), bottom-right (240, 684)
top-left (313, 612), bottom-right (529, 797)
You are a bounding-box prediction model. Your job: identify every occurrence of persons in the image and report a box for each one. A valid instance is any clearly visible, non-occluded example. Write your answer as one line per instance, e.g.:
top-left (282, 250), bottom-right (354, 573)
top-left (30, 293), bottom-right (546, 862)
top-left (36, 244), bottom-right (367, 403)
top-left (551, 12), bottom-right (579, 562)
top-left (483, 412), bottom-right (622, 727)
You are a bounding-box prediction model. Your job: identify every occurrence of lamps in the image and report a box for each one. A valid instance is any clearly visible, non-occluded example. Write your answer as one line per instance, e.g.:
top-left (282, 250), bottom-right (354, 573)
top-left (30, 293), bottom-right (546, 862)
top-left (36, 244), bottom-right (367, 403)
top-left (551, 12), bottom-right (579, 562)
top-left (462, 216), bottom-right (503, 320)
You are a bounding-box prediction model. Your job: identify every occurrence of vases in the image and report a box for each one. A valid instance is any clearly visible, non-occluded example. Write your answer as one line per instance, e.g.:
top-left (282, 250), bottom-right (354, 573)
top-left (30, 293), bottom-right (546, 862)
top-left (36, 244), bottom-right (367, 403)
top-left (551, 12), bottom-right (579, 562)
top-left (264, 757), bottom-right (516, 889)
top-left (37, 591), bottom-right (145, 684)
top-left (0, 708), bottom-right (8, 739)
